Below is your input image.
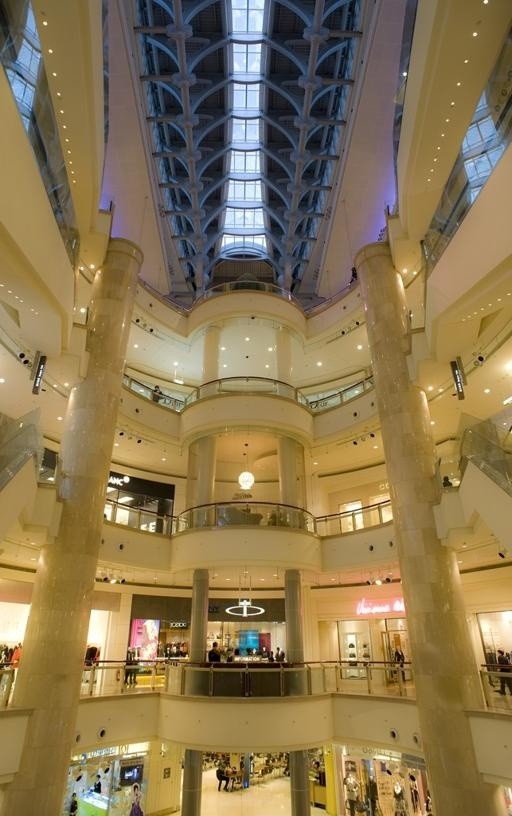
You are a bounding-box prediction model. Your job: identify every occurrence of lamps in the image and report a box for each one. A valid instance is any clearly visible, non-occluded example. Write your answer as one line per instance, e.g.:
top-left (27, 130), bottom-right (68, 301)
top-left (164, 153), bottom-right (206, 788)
top-left (237, 452), bottom-right (255, 490)
top-left (224, 573), bottom-right (266, 618)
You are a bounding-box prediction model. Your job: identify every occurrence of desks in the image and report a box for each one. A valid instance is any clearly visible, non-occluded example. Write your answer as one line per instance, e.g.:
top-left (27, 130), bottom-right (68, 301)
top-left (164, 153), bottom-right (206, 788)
top-left (220, 761), bottom-right (289, 792)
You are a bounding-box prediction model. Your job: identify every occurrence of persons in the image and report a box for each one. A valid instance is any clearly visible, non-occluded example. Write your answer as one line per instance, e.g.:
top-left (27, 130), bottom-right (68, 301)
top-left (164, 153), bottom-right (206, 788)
top-left (158, 640), bottom-right (286, 664)
top-left (1, 641), bottom-right (23, 667)
top-left (366, 776), bottom-right (379, 816)
top-left (129, 785), bottom-right (144, 816)
top-left (491, 648), bottom-right (512, 696)
top-left (392, 782), bottom-right (406, 816)
top-left (69, 793), bottom-right (79, 816)
top-left (393, 645), bottom-right (406, 682)
top-left (283, 759), bottom-right (290, 777)
top-left (313, 762), bottom-right (322, 780)
top-left (151, 385), bottom-right (162, 403)
top-left (441, 475), bottom-right (452, 487)
top-left (230, 756), bottom-right (244, 792)
top-left (345, 774), bottom-right (362, 816)
top-left (215, 764), bottom-right (229, 793)
top-left (123, 647), bottom-right (140, 686)
top-left (93, 775), bottom-right (102, 794)
